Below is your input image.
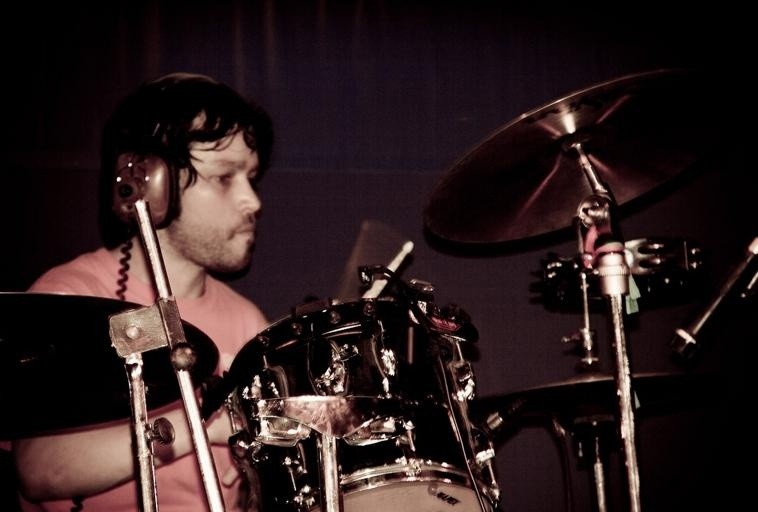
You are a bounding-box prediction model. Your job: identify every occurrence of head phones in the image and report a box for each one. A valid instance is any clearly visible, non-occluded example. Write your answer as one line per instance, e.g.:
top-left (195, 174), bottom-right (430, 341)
top-left (111, 151), bottom-right (181, 232)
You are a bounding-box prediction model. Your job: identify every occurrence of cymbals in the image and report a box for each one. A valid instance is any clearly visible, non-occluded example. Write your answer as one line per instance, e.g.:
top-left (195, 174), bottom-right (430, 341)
top-left (466, 371), bottom-right (689, 416)
top-left (425, 69), bottom-right (724, 243)
top-left (1, 291), bottom-right (219, 442)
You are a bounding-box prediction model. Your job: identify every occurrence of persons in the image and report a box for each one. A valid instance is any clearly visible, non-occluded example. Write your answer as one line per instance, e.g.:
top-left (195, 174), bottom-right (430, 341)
top-left (9, 73), bottom-right (273, 512)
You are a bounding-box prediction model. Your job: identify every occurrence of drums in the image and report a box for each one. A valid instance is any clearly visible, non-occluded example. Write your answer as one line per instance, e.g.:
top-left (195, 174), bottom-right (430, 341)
top-left (224, 278), bottom-right (500, 512)
